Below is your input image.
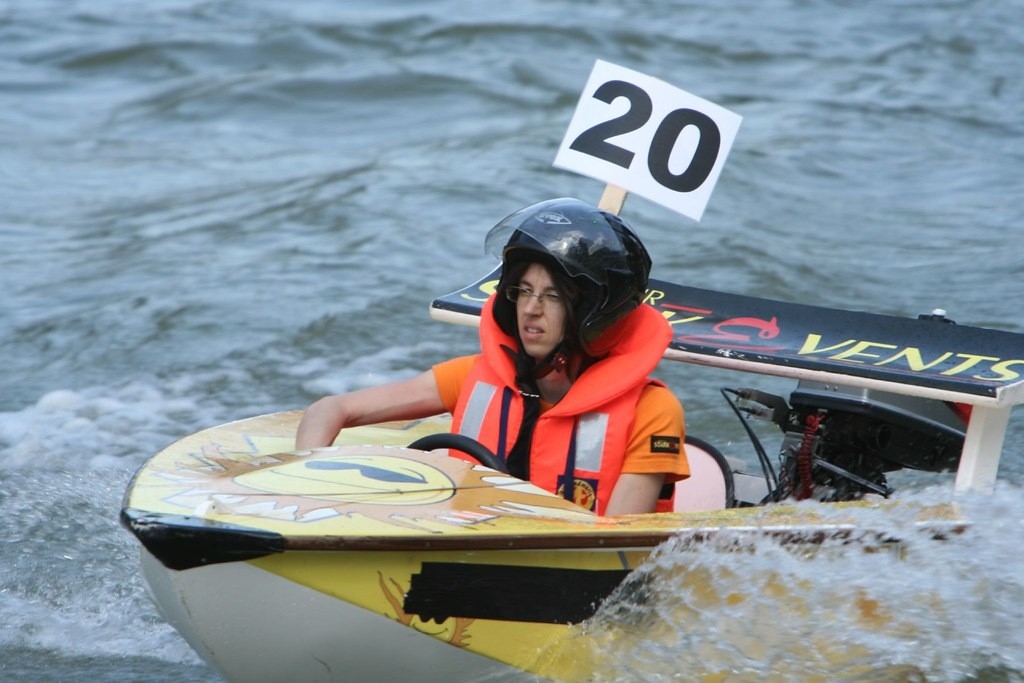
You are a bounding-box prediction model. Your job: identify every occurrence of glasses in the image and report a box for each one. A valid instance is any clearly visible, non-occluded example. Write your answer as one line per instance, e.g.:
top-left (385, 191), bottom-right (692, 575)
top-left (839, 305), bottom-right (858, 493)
top-left (504, 284), bottom-right (564, 304)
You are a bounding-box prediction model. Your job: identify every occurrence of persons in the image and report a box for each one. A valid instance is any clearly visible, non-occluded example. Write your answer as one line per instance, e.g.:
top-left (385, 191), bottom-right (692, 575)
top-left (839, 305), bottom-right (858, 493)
top-left (295, 197), bottom-right (691, 519)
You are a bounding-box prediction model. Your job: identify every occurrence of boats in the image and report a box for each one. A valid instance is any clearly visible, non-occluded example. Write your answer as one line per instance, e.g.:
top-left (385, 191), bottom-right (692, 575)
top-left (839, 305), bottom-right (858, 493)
top-left (120, 260), bottom-right (1024, 683)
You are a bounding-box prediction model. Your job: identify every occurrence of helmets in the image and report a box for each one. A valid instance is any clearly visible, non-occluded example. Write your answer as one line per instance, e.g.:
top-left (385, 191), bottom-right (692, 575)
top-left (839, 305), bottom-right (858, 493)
top-left (485, 197), bottom-right (651, 351)
